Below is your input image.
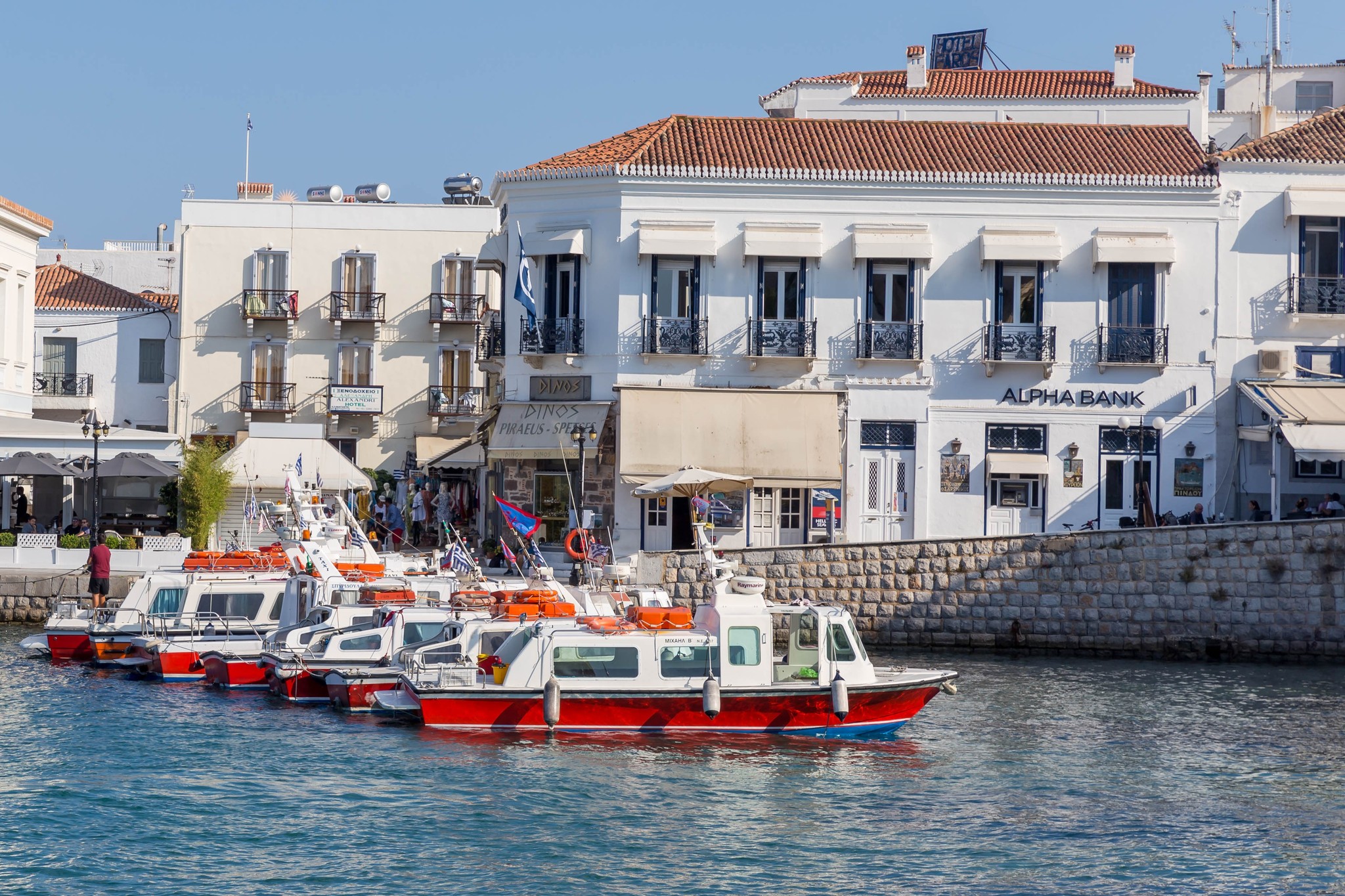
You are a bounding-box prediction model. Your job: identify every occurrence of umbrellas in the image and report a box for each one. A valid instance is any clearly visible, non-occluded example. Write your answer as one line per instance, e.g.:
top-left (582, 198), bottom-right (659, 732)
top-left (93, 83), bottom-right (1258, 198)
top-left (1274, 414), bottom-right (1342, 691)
top-left (631, 465), bottom-right (754, 550)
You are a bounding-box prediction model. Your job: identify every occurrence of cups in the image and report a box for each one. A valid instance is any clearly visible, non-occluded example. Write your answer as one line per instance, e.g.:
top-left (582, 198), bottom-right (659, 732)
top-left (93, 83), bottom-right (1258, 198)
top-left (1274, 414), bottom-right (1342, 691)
top-left (113, 518), bottom-right (118, 525)
top-left (1213, 514), bottom-right (1216, 520)
top-left (133, 528), bottom-right (139, 536)
top-left (1230, 515), bottom-right (1234, 520)
top-left (151, 527), bottom-right (155, 531)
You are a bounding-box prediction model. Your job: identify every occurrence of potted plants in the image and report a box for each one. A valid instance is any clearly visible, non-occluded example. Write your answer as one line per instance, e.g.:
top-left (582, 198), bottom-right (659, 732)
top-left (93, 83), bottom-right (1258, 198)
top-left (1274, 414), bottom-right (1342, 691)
top-left (481, 538), bottom-right (497, 559)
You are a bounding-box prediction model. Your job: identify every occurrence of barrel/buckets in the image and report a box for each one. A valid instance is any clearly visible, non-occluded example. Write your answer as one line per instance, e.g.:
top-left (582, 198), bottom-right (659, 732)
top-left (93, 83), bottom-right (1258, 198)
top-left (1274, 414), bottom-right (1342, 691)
top-left (492, 663), bottom-right (510, 684)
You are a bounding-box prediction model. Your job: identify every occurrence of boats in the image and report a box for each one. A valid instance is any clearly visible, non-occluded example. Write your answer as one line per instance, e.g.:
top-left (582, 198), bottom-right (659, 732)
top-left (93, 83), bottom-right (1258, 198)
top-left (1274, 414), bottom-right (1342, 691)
top-left (41, 439), bottom-right (960, 743)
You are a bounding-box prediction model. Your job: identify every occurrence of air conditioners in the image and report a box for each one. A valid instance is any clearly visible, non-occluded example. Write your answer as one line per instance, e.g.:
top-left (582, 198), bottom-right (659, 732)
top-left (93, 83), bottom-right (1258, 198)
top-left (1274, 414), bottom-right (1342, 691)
top-left (1257, 350), bottom-right (1291, 373)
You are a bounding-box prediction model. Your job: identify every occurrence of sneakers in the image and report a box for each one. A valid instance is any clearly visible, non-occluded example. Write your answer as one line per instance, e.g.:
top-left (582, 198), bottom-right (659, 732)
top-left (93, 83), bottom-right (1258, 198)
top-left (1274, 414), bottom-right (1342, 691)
top-left (503, 569), bottom-right (513, 575)
top-left (518, 570), bottom-right (522, 576)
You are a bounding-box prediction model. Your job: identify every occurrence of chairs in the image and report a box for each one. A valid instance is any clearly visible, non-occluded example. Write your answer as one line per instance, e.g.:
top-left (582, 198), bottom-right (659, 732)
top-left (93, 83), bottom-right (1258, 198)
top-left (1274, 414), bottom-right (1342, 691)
top-left (1119, 517), bottom-right (1136, 528)
top-left (1176, 507), bottom-right (1345, 525)
top-left (7, 527), bottom-right (180, 549)
top-left (243, 292), bottom-right (482, 414)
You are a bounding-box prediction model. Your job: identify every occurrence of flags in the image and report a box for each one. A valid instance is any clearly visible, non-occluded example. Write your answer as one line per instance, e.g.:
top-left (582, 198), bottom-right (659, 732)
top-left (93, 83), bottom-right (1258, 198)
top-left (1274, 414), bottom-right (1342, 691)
top-left (440, 544), bottom-right (454, 569)
top-left (225, 453), bottom-right (324, 554)
top-left (495, 497), bottom-right (608, 568)
top-left (453, 540), bottom-right (469, 575)
top-left (513, 221), bottom-right (537, 335)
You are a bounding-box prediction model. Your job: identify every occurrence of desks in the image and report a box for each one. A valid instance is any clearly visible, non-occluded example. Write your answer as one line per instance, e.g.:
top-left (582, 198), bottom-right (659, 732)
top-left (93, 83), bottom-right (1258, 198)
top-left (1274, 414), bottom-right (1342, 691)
top-left (453, 535), bottom-right (480, 548)
top-left (57, 534), bottom-right (67, 548)
top-left (537, 517), bottom-right (569, 539)
top-left (1280, 512), bottom-right (1322, 520)
top-left (98, 517), bottom-right (175, 549)
top-left (1214, 520), bottom-right (1254, 523)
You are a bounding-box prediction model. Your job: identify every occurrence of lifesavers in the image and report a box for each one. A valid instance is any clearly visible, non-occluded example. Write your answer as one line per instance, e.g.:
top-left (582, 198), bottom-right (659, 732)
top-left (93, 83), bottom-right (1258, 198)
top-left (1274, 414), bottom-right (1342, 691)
top-left (226, 551), bottom-right (261, 558)
top-left (188, 552), bottom-right (225, 558)
top-left (452, 593), bottom-right (497, 605)
top-left (512, 590), bottom-right (558, 597)
top-left (359, 585), bottom-right (411, 593)
top-left (565, 528), bottom-right (595, 560)
top-left (576, 616), bottom-right (623, 624)
top-left (587, 618), bottom-right (636, 634)
top-left (451, 602), bottom-right (494, 611)
top-left (513, 596), bottom-right (559, 603)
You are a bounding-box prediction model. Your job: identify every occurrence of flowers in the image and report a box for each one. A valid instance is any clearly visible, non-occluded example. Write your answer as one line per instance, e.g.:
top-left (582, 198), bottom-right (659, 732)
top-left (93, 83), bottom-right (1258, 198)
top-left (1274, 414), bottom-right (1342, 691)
top-left (495, 545), bottom-right (517, 555)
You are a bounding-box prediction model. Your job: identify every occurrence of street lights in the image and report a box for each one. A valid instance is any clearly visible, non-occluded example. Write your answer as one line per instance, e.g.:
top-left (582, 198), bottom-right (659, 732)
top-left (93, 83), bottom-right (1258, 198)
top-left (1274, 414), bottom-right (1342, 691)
top-left (80, 418), bottom-right (110, 553)
top-left (1117, 413), bottom-right (1166, 528)
top-left (568, 423), bottom-right (599, 584)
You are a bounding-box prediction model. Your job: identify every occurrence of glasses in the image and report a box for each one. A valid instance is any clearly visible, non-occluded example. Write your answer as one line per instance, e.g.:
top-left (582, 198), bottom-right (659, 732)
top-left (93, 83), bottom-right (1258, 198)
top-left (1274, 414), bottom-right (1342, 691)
top-left (81, 522), bottom-right (86, 524)
top-left (72, 519), bottom-right (79, 522)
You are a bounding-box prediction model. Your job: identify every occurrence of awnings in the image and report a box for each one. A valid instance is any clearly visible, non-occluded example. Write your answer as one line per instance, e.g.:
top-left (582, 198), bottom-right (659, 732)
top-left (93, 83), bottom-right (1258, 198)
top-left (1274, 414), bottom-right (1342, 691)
top-left (414, 433), bottom-right (485, 470)
top-left (1238, 380), bottom-right (1345, 463)
top-left (486, 401), bottom-right (611, 460)
top-left (615, 386), bottom-right (844, 490)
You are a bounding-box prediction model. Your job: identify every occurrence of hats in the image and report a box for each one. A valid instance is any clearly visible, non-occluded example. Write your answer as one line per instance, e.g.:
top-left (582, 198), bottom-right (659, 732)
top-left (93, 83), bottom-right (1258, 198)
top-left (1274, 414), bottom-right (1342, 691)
top-left (378, 495), bottom-right (386, 502)
top-left (72, 517), bottom-right (79, 520)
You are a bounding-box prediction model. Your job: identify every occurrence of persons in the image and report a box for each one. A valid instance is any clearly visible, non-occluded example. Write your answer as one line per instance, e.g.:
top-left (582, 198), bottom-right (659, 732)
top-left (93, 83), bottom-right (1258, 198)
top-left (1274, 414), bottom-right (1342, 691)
top-left (332, 504), bottom-right (335, 514)
top-left (371, 512), bottom-right (388, 552)
top-left (402, 482), bottom-right (455, 547)
top-left (11, 487), bottom-right (90, 538)
top-left (384, 498), bottom-right (405, 552)
top-left (372, 495), bottom-right (386, 524)
top-left (87, 534), bottom-right (111, 622)
top-left (354, 488), bottom-right (372, 535)
top-left (504, 537), bottom-right (536, 576)
top-left (1247, 500), bottom-right (1264, 521)
top-left (382, 483), bottom-right (396, 504)
top-left (1287, 492), bottom-right (1345, 520)
top-left (1188, 503), bottom-right (1206, 525)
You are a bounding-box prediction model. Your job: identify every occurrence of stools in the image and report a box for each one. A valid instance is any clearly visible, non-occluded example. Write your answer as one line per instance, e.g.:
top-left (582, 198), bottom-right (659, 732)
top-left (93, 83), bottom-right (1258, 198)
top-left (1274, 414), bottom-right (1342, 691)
top-left (560, 528), bottom-right (570, 542)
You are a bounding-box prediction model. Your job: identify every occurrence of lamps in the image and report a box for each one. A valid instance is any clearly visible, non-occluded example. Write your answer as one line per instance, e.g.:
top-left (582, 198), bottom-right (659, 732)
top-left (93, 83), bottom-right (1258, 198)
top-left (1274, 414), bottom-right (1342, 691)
top-left (157, 505), bottom-right (168, 526)
top-left (951, 438), bottom-right (962, 453)
top-left (517, 462), bottom-right (523, 473)
top-left (208, 242), bottom-right (462, 434)
top-left (1261, 411), bottom-right (1268, 420)
top-left (1276, 432), bottom-right (1284, 444)
top-left (124, 419), bottom-right (131, 425)
top-left (1068, 442), bottom-right (1079, 457)
top-left (1184, 441), bottom-right (1196, 456)
top-left (496, 461), bottom-right (504, 473)
top-left (594, 464), bottom-right (600, 475)
